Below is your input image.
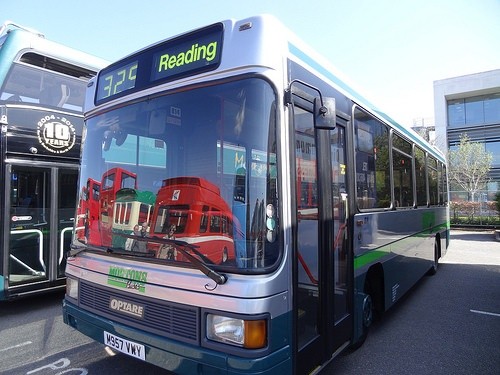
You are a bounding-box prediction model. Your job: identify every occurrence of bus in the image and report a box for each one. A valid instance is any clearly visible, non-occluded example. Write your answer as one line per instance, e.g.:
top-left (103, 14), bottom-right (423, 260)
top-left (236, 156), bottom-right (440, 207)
top-left (0, 21), bottom-right (117, 301)
top-left (61, 12), bottom-right (451, 375)
top-left (77, 167), bottom-right (241, 266)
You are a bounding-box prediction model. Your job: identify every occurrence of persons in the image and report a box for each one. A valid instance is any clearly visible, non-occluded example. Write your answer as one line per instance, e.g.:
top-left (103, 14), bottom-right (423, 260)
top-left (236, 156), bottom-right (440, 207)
top-left (125, 225), bottom-right (140, 251)
top-left (157, 224), bottom-right (177, 260)
top-left (84, 209), bottom-right (91, 243)
top-left (131, 222), bottom-right (149, 253)
